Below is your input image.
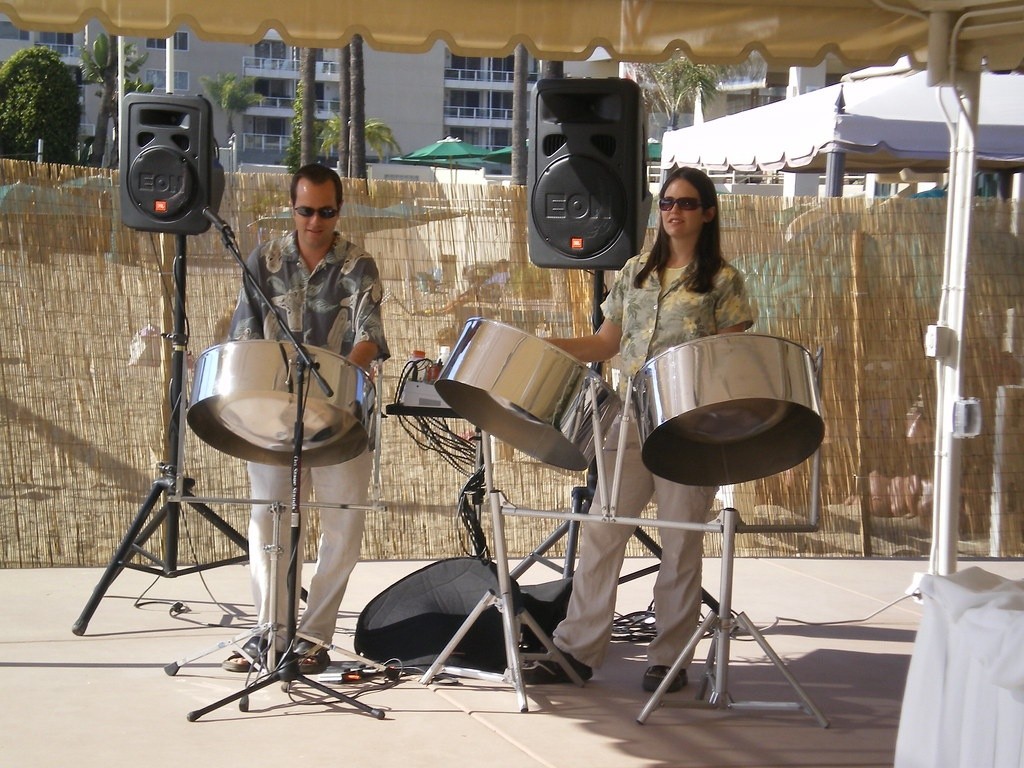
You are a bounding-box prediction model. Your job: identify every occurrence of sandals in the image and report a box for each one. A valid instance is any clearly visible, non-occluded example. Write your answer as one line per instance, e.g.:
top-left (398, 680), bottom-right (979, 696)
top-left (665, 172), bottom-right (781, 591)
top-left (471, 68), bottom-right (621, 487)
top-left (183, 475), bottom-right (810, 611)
top-left (223, 635), bottom-right (284, 672)
top-left (293, 638), bottom-right (329, 674)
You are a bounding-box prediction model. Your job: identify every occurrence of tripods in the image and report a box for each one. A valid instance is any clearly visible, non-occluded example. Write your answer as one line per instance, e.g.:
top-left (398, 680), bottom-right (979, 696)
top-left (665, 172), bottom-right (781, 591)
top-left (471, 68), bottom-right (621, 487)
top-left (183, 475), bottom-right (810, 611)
top-left (187, 235), bottom-right (386, 725)
top-left (72, 235), bottom-right (309, 636)
top-left (507, 269), bottom-right (725, 621)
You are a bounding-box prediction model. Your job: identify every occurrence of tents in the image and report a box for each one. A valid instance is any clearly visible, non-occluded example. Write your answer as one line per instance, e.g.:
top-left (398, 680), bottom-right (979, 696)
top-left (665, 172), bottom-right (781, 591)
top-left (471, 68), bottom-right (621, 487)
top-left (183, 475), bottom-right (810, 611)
top-left (655, 54), bottom-right (1024, 193)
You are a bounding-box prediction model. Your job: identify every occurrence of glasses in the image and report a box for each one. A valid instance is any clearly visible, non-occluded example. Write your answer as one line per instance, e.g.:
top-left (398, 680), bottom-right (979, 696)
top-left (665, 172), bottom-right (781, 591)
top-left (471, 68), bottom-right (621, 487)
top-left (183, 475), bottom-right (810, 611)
top-left (659, 197), bottom-right (705, 211)
top-left (293, 201), bottom-right (341, 218)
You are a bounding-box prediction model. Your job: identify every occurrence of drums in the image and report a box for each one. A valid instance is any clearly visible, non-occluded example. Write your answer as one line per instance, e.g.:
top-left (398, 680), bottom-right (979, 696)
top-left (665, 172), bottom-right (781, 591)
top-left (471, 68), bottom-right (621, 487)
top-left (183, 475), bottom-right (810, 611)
top-left (631, 332), bottom-right (826, 487)
top-left (433, 317), bottom-right (624, 472)
top-left (185, 339), bottom-right (377, 468)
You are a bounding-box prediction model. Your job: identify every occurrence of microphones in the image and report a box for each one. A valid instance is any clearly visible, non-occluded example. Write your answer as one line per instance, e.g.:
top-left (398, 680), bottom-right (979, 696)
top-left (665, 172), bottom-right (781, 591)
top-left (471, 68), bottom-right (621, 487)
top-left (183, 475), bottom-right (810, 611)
top-left (201, 204), bottom-right (236, 240)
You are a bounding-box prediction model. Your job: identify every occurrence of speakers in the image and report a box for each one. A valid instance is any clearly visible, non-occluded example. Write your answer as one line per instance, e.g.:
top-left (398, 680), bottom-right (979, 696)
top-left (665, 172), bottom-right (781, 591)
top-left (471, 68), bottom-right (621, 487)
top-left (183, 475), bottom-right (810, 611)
top-left (118, 93), bottom-right (225, 236)
top-left (527, 76), bottom-right (653, 270)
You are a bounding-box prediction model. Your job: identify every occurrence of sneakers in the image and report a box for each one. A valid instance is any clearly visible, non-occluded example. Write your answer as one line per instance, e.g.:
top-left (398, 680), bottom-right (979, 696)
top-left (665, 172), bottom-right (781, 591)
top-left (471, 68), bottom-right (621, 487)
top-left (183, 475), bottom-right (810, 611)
top-left (643, 665), bottom-right (688, 693)
top-left (503, 652), bottom-right (593, 686)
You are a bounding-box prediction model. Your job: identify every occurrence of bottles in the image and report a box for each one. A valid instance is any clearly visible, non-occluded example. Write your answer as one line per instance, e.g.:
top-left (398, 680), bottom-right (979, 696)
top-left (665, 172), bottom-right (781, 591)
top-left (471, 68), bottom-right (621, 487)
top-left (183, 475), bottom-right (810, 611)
top-left (436, 346), bottom-right (451, 366)
top-left (404, 350), bottom-right (426, 381)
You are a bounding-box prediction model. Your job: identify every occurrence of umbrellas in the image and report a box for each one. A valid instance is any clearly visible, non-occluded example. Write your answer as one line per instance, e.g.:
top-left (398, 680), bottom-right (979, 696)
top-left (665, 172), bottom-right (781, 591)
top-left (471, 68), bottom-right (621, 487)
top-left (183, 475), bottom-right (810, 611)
top-left (390, 135), bottom-right (660, 190)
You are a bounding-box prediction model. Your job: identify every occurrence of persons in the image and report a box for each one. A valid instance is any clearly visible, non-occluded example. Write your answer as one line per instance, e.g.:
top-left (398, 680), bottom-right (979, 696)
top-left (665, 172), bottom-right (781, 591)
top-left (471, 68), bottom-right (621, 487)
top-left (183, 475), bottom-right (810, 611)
top-left (219, 165), bottom-right (392, 674)
top-left (502, 171), bottom-right (755, 686)
